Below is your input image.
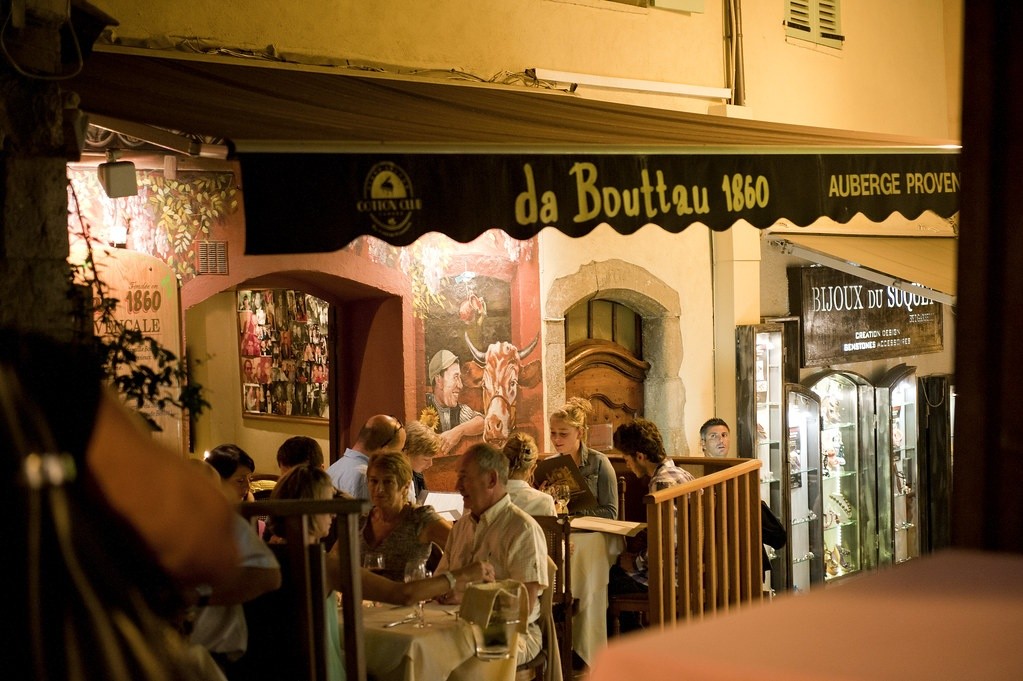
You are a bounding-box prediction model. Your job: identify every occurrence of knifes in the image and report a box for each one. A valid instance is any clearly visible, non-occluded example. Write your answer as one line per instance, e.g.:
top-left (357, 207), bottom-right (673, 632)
top-left (384, 617), bottom-right (417, 628)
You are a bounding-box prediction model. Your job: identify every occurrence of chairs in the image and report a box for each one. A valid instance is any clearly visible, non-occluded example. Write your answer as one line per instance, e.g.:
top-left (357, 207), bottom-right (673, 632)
top-left (245, 473), bottom-right (717, 681)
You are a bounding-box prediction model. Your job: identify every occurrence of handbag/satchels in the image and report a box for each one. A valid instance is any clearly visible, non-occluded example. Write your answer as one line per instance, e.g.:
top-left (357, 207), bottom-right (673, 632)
top-left (26, 443), bottom-right (227, 680)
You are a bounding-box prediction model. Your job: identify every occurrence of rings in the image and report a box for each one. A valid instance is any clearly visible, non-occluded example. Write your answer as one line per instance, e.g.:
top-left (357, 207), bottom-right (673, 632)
top-left (487, 571), bottom-right (491, 576)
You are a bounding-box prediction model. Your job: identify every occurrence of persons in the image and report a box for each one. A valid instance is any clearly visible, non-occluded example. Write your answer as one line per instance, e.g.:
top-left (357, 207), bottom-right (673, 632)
top-left (264, 464), bottom-right (496, 681)
top-left (699, 418), bottom-right (788, 604)
top-left (537, 397), bottom-right (618, 521)
top-left (233, 283), bottom-right (332, 421)
top-left (433, 443), bottom-right (549, 681)
top-left (0, 329), bottom-right (281, 680)
top-left (497, 431), bottom-right (575, 584)
top-left (205, 444), bottom-right (260, 570)
top-left (144, 458), bottom-right (284, 681)
top-left (275, 414), bottom-right (454, 606)
top-left (612, 418), bottom-right (716, 627)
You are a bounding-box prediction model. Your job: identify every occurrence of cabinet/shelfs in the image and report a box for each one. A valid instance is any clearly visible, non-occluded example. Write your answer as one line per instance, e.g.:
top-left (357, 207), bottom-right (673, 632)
top-left (783, 360), bottom-right (920, 597)
top-left (734, 323), bottom-right (788, 600)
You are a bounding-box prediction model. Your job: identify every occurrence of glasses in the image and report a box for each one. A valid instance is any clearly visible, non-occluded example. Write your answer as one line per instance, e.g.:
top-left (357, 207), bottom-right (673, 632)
top-left (381, 414), bottom-right (404, 448)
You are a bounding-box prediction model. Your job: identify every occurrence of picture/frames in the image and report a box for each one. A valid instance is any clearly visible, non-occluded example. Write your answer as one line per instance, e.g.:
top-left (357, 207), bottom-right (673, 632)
top-left (235, 286), bottom-right (331, 426)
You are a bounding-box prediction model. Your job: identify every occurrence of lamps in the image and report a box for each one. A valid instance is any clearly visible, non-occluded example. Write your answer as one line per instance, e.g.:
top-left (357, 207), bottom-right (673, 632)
top-left (769, 238), bottom-right (957, 308)
top-left (525, 68), bottom-right (732, 100)
top-left (97, 148), bottom-right (137, 198)
top-left (62, 90), bottom-right (89, 162)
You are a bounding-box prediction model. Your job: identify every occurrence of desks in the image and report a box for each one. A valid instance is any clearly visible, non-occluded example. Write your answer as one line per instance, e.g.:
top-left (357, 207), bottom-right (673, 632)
top-left (335, 599), bottom-right (520, 681)
top-left (556, 533), bottom-right (625, 669)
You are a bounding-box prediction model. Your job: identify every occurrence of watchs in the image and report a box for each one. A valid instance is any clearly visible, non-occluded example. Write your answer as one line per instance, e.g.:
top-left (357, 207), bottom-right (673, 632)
top-left (196, 592), bottom-right (210, 612)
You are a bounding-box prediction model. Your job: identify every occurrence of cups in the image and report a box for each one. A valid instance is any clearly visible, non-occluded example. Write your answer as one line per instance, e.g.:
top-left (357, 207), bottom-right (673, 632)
top-left (542, 486), bottom-right (556, 502)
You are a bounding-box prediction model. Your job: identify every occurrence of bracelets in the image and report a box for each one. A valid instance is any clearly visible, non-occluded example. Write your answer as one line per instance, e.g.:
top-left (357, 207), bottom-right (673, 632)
top-left (440, 570), bottom-right (456, 591)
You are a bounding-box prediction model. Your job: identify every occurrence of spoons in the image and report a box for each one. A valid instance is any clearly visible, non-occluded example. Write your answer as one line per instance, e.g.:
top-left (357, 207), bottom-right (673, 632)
top-left (442, 605), bottom-right (461, 615)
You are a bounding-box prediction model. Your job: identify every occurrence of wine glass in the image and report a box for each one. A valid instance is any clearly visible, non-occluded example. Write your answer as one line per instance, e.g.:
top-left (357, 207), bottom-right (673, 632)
top-left (409, 568), bottom-right (432, 628)
top-left (556, 485), bottom-right (570, 513)
top-left (363, 553), bottom-right (386, 610)
top-left (405, 559), bottom-right (427, 617)
